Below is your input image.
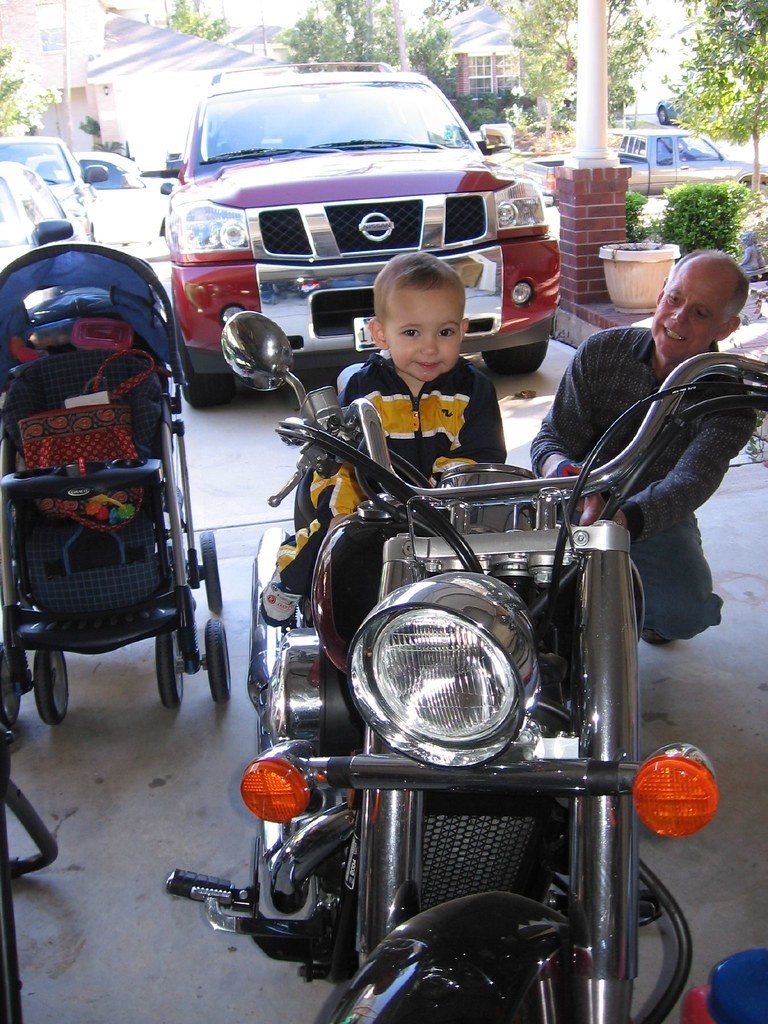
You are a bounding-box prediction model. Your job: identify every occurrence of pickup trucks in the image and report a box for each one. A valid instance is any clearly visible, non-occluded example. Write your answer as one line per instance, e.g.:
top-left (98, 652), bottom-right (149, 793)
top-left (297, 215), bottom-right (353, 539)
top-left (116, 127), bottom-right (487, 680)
top-left (522, 128), bottom-right (767, 215)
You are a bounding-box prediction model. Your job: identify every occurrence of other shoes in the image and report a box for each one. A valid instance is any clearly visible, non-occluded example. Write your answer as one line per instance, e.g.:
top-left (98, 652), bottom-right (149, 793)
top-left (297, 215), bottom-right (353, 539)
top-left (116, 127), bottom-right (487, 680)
top-left (641, 627), bottom-right (671, 644)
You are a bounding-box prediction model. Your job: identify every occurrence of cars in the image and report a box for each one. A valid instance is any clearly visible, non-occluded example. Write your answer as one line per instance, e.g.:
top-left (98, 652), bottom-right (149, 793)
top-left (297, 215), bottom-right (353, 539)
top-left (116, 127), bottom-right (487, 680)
top-left (28, 151), bottom-right (180, 245)
top-left (1, 160), bottom-right (92, 268)
top-left (656, 90), bottom-right (693, 125)
top-left (0, 134), bottom-right (109, 243)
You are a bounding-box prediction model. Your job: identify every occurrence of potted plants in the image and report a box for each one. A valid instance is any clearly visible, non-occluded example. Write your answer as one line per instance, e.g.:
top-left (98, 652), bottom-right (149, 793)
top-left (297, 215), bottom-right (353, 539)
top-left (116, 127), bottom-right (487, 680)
top-left (599, 237), bottom-right (682, 313)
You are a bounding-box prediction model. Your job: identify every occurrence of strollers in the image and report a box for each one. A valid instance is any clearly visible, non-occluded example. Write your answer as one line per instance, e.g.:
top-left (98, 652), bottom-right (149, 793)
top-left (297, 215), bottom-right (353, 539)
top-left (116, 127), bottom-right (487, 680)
top-left (1, 242), bottom-right (231, 726)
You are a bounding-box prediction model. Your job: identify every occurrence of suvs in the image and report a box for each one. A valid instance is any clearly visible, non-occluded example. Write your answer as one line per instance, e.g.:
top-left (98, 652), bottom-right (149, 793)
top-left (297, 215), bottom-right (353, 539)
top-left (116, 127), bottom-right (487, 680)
top-left (156, 62), bottom-right (563, 409)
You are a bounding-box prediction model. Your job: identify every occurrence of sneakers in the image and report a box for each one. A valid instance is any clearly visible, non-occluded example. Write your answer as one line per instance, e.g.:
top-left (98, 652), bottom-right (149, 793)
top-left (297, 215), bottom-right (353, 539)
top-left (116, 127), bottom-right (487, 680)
top-left (261, 568), bottom-right (300, 626)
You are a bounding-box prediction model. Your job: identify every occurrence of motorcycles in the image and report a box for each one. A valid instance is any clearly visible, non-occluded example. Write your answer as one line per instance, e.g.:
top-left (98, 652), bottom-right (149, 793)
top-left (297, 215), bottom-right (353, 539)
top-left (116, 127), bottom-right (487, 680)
top-left (163, 312), bottom-right (767, 1023)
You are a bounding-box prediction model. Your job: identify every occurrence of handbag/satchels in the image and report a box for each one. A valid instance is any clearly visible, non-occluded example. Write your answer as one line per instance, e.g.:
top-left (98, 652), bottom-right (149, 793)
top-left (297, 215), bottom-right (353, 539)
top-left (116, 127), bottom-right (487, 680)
top-left (17, 349), bottom-right (156, 531)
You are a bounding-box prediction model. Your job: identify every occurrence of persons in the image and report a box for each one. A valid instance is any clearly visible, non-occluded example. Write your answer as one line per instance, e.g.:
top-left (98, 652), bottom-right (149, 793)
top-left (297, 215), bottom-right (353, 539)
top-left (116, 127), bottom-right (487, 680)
top-left (529, 249), bottom-right (756, 644)
top-left (259, 252), bottom-right (507, 626)
